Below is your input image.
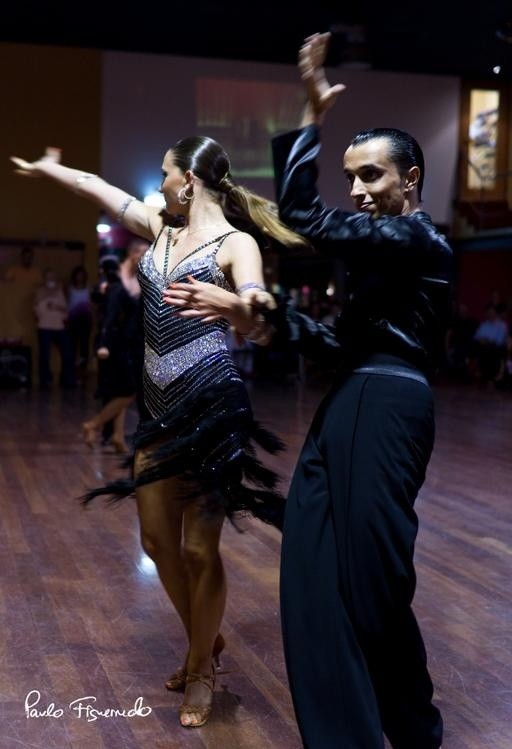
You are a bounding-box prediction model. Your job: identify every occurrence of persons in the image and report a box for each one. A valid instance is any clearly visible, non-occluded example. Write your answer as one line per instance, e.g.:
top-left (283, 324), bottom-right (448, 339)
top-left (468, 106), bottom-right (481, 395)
top-left (469, 302), bottom-right (508, 385)
top-left (62, 265), bottom-right (95, 391)
top-left (31, 265), bottom-right (77, 403)
top-left (8, 132), bottom-right (273, 729)
top-left (320, 300), bottom-right (343, 327)
top-left (308, 302), bottom-right (321, 322)
top-left (88, 237), bottom-right (154, 447)
top-left (175, 30), bottom-right (458, 747)
top-left (79, 253), bottom-right (144, 455)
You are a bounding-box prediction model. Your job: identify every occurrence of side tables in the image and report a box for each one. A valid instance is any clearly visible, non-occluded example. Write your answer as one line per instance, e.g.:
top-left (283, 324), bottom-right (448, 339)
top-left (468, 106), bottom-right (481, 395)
top-left (0, 344), bottom-right (32, 388)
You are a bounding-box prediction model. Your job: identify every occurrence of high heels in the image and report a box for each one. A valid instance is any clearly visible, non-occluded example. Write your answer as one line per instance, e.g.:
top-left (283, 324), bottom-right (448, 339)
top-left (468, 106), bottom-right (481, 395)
top-left (166, 634), bottom-right (224, 689)
top-left (110, 435), bottom-right (130, 453)
top-left (179, 659), bottom-right (216, 727)
top-left (77, 423), bottom-right (98, 450)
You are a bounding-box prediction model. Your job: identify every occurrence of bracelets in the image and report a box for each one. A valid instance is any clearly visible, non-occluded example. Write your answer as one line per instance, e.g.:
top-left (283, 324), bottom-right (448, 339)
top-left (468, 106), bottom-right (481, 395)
top-left (69, 172), bottom-right (98, 196)
top-left (238, 312), bottom-right (265, 338)
top-left (243, 324), bottom-right (276, 345)
